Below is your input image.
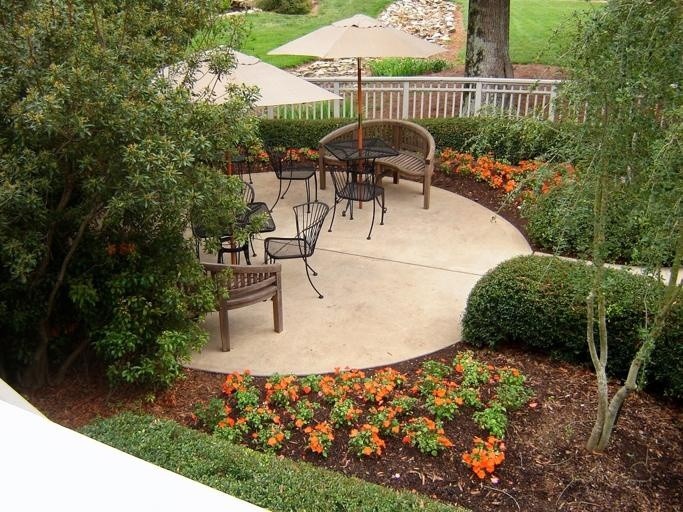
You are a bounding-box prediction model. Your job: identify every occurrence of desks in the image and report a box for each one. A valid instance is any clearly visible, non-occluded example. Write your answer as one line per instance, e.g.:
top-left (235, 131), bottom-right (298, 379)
top-left (323, 140), bottom-right (398, 211)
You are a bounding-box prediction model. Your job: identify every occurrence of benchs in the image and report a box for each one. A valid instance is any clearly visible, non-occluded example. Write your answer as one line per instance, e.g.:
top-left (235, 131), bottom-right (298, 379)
top-left (317, 119), bottom-right (436, 210)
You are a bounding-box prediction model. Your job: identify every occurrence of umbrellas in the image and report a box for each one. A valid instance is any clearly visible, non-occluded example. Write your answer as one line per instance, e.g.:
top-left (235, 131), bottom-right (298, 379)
top-left (268, 13), bottom-right (450, 210)
top-left (151, 46), bottom-right (344, 265)
